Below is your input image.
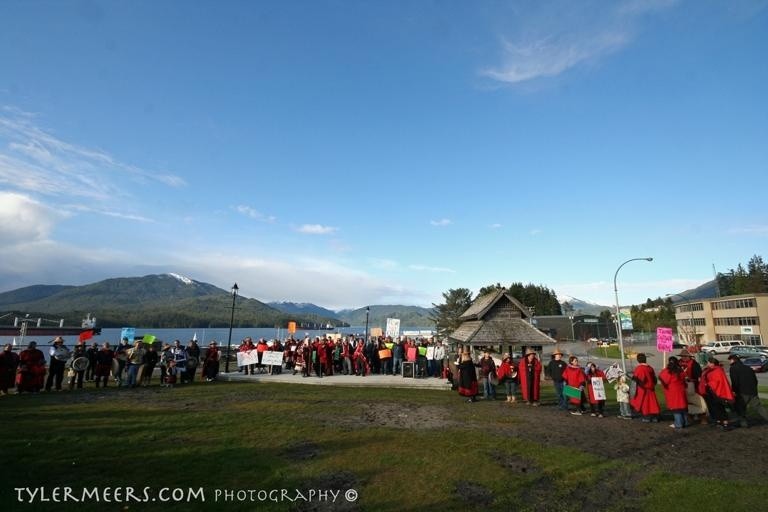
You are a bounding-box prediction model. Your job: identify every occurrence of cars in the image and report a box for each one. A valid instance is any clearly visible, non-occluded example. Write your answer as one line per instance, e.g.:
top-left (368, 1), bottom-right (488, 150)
top-left (741, 356), bottom-right (767, 371)
top-left (587, 336), bottom-right (637, 345)
top-left (431, 331), bottom-right (436, 337)
top-left (756, 346), bottom-right (767, 353)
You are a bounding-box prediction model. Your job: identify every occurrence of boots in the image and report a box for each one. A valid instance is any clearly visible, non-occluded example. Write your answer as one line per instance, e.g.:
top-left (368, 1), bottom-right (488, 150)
top-left (503, 395), bottom-right (518, 403)
top-left (687, 415), bottom-right (696, 424)
top-left (700, 415), bottom-right (709, 425)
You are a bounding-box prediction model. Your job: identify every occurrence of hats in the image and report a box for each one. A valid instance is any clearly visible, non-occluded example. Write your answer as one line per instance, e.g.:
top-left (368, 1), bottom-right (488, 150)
top-left (209, 341), bottom-right (216, 345)
top-left (551, 349), bottom-right (562, 355)
top-left (675, 350), bottom-right (694, 357)
top-left (503, 354), bottom-right (510, 361)
top-left (524, 349), bottom-right (536, 356)
top-left (55, 337), bottom-right (64, 343)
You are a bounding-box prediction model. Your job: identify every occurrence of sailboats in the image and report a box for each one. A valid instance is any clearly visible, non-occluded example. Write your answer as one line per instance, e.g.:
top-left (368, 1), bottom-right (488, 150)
top-left (325, 319), bottom-right (335, 330)
top-left (319, 320), bottom-right (326, 330)
top-left (312, 322), bottom-right (318, 330)
top-left (304, 322), bottom-right (313, 330)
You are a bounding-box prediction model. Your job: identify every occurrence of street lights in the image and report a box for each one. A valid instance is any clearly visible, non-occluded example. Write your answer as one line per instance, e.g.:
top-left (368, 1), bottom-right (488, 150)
top-left (665, 294), bottom-right (699, 355)
top-left (224, 281), bottom-right (241, 373)
top-left (435, 316), bottom-right (440, 339)
top-left (614, 257), bottom-right (653, 374)
top-left (611, 314), bottom-right (618, 338)
top-left (571, 320), bottom-right (581, 342)
top-left (363, 305), bottom-right (371, 342)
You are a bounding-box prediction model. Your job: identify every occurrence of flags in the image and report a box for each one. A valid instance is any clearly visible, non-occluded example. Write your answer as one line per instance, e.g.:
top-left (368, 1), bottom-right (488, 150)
top-left (78, 330), bottom-right (94, 344)
top-left (142, 334), bottom-right (157, 345)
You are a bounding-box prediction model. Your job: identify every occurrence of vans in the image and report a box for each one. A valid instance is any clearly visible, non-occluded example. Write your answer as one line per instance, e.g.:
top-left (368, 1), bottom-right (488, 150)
top-left (729, 345), bottom-right (767, 359)
top-left (701, 340), bottom-right (745, 355)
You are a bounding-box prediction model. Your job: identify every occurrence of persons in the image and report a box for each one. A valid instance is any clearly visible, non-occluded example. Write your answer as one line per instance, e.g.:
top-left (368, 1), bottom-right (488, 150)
top-left (236, 333), bottom-right (447, 378)
top-left (114, 337), bottom-right (222, 389)
top-left (67, 340), bottom-right (87, 390)
top-left (17, 340), bottom-right (47, 396)
top-left (0, 343), bottom-right (19, 396)
top-left (86, 342), bottom-right (99, 381)
top-left (46, 337), bottom-right (69, 393)
top-left (95, 341), bottom-right (117, 390)
top-left (454, 347), bottom-right (760, 430)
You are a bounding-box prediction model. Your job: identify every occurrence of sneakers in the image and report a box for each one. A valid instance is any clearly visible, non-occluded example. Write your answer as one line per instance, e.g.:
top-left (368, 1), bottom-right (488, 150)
top-left (712, 420), bottom-right (721, 428)
top-left (721, 424), bottom-right (728, 432)
top-left (570, 411), bottom-right (632, 420)
